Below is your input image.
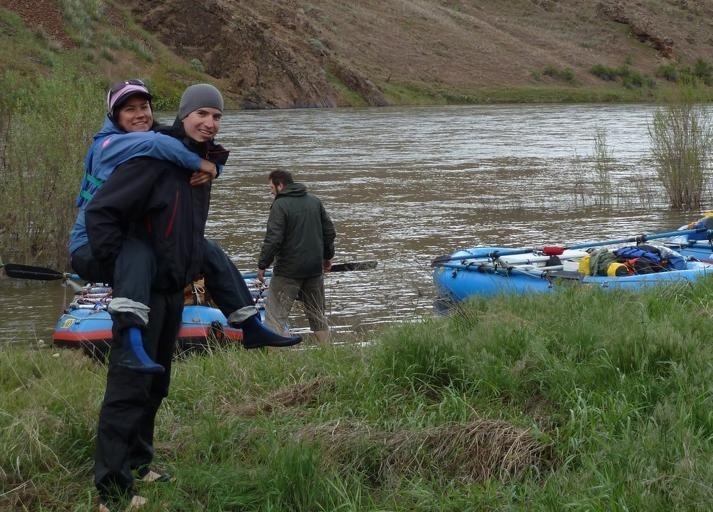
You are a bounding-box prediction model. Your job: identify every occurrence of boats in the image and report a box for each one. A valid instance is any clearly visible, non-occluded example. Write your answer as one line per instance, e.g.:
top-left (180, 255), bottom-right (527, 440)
top-left (53, 283), bottom-right (293, 352)
top-left (434, 213), bottom-right (713, 307)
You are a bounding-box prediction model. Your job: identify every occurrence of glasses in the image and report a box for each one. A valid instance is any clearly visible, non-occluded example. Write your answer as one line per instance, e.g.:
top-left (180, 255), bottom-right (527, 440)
top-left (108, 79), bottom-right (145, 106)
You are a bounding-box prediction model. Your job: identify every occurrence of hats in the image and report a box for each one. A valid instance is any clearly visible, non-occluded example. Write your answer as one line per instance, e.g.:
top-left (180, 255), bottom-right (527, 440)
top-left (107, 78), bottom-right (150, 114)
top-left (178, 84), bottom-right (225, 121)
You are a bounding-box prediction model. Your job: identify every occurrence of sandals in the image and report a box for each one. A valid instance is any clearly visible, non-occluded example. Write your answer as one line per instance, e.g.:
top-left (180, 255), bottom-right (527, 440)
top-left (133, 464), bottom-right (178, 488)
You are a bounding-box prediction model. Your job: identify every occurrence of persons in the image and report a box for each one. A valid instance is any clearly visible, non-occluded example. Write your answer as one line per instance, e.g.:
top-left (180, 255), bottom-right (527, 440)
top-left (76, 78), bottom-right (232, 510)
top-left (62, 77), bottom-right (305, 375)
top-left (257, 169), bottom-right (337, 344)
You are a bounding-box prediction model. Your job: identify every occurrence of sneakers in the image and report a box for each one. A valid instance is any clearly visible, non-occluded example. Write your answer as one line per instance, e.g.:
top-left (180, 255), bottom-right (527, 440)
top-left (112, 327), bottom-right (167, 375)
top-left (243, 323), bottom-right (303, 350)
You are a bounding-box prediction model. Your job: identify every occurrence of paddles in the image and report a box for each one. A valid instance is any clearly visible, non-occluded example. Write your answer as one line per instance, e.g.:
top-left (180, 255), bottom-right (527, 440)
top-left (438, 262), bottom-right (586, 287)
top-left (241, 260), bottom-right (377, 278)
top-left (4, 263), bottom-right (79, 280)
top-left (430, 248), bottom-right (544, 267)
